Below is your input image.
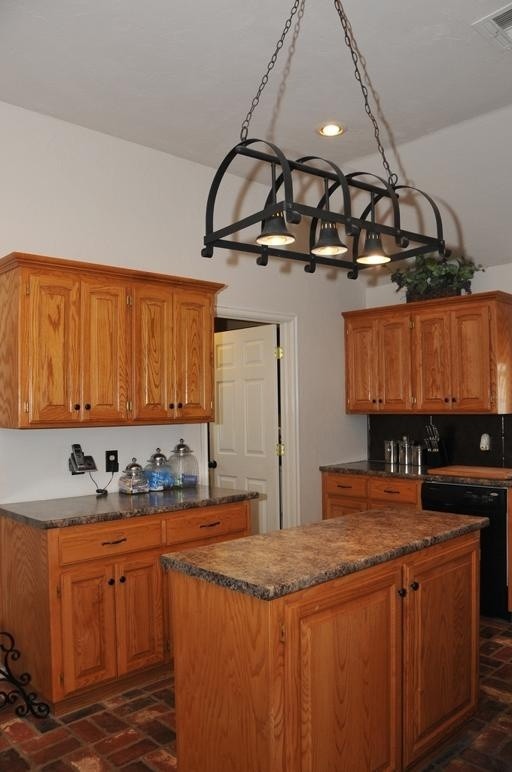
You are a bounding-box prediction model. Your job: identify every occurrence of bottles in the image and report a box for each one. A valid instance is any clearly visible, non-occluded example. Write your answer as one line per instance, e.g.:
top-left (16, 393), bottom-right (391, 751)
top-left (119, 438), bottom-right (198, 495)
top-left (385, 436), bottom-right (425, 466)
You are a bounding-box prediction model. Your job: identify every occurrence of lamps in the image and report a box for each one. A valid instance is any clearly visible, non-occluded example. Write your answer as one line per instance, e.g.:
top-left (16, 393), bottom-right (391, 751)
top-left (197, 2), bottom-right (450, 280)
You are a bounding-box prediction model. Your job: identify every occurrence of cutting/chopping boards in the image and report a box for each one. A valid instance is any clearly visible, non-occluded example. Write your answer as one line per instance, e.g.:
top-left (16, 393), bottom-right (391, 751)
top-left (426, 465), bottom-right (512, 480)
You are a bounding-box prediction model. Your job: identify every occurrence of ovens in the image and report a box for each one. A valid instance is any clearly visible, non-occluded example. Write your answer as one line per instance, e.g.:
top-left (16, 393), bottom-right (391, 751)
top-left (422, 483), bottom-right (508, 612)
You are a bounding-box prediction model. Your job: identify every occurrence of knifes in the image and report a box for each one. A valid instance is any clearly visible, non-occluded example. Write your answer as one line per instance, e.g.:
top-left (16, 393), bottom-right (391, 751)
top-left (424, 424), bottom-right (441, 452)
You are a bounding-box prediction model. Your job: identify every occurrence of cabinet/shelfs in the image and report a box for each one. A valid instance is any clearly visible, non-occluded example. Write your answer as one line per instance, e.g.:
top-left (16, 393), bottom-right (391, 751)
top-left (412, 304), bottom-right (494, 413)
top-left (279, 538), bottom-right (480, 772)
top-left (58, 520), bottom-right (165, 697)
top-left (343, 309), bottom-right (412, 412)
top-left (23, 267), bottom-right (131, 424)
top-left (161, 504), bottom-right (248, 547)
top-left (320, 473), bottom-right (418, 519)
top-left (132, 279), bottom-right (213, 422)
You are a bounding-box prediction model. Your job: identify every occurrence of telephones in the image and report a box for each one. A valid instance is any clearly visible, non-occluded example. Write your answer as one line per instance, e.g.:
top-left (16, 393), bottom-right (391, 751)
top-left (68, 443), bottom-right (97, 476)
